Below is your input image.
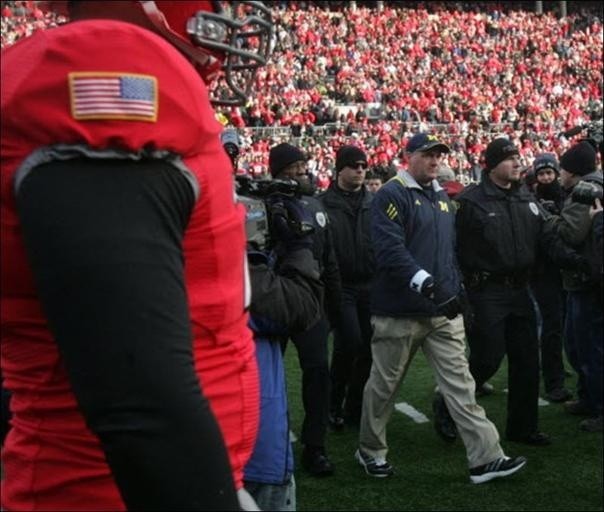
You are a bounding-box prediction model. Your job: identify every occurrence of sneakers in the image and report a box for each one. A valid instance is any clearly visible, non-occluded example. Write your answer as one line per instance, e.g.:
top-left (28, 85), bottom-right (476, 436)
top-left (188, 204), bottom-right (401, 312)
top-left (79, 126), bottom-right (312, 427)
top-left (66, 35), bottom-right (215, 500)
top-left (470, 455), bottom-right (526, 484)
top-left (510, 430), bottom-right (549, 445)
top-left (355, 449), bottom-right (392, 479)
top-left (546, 387), bottom-right (604, 432)
top-left (480, 382), bottom-right (492, 391)
top-left (304, 409), bottom-right (345, 473)
top-left (432, 398), bottom-right (455, 440)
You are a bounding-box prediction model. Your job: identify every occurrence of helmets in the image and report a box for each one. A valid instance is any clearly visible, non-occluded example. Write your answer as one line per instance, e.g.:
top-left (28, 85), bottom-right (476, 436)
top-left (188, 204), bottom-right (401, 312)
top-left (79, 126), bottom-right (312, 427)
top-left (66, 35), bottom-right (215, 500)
top-left (67, 0), bottom-right (272, 105)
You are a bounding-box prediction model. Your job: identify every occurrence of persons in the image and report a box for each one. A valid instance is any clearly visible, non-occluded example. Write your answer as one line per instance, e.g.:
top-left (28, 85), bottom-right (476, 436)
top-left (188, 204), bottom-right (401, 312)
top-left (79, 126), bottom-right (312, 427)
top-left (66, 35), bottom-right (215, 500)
top-left (432, 139), bottom-right (590, 445)
top-left (589, 199), bottom-right (604, 274)
top-left (353, 133), bottom-right (527, 485)
top-left (0, 0), bottom-right (603, 197)
top-left (546, 141), bottom-right (604, 432)
top-left (316, 145), bottom-right (376, 431)
top-left (0, 0), bottom-right (272, 512)
top-left (530, 153), bottom-right (573, 402)
top-left (237, 195), bottom-right (325, 512)
top-left (268, 143), bottom-right (344, 476)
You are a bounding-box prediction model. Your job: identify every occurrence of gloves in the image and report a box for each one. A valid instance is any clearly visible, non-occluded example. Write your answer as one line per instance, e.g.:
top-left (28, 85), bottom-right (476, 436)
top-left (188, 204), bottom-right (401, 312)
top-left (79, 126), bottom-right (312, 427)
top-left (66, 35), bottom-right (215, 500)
top-left (422, 278), bottom-right (459, 319)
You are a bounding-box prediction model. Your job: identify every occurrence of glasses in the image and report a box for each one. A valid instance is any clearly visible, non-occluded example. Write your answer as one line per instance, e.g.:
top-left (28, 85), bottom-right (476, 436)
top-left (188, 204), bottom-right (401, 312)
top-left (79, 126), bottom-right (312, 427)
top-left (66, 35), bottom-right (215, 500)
top-left (345, 161), bottom-right (367, 169)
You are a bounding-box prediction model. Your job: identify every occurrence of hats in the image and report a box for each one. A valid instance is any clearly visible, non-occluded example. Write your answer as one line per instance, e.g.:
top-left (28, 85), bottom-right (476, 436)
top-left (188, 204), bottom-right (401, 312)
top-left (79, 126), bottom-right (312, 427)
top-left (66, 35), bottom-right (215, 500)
top-left (407, 134), bottom-right (448, 153)
top-left (485, 138), bottom-right (518, 168)
top-left (269, 142), bottom-right (304, 178)
top-left (536, 141), bottom-right (596, 175)
top-left (335, 146), bottom-right (365, 171)
